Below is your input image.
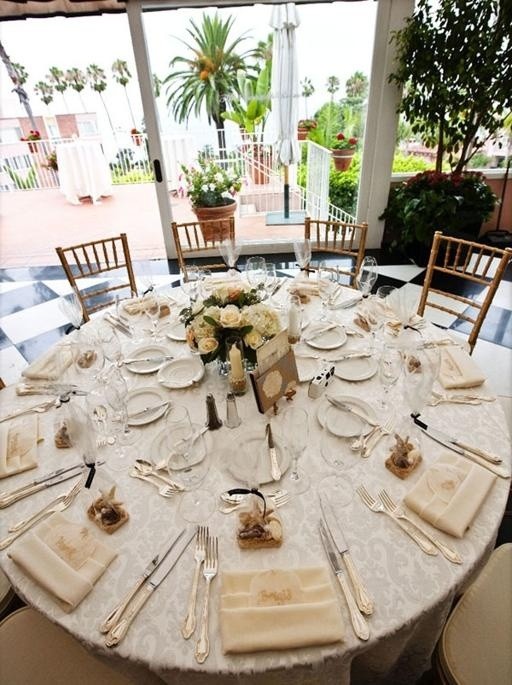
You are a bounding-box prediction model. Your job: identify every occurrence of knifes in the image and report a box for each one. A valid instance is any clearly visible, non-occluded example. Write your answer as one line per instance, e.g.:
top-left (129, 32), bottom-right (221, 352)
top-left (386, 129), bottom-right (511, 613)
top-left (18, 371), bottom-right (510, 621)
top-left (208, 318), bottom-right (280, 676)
top-left (0, 461), bottom-right (83, 508)
top-left (113, 400), bottom-right (172, 422)
top-left (106, 525), bottom-right (188, 648)
top-left (329, 322), bottom-right (363, 339)
top-left (325, 394), bottom-right (378, 427)
top-left (316, 518), bottom-right (370, 642)
top-left (316, 491), bottom-right (372, 614)
top-left (104, 310), bottom-right (137, 335)
top-left (100, 317), bottom-right (136, 340)
top-left (15, 390), bottom-right (87, 397)
top-left (271, 276), bottom-right (289, 297)
top-left (123, 357), bottom-right (175, 363)
top-left (265, 424), bottom-right (281, 481)
top-left (0, 463), bottom-right (80, 499)
top-left (18, 382), bottom-right (78, 391)
top-left (8, 496), bottom-right (64, 532)
top-left (324, 353), bottom-right (371, 364)
top-left (413, 416), bottom-right (502, 464)
top-left (415, 423), bottom-right (511, 478)
top-left (99, 528), bottom-right (184, 632)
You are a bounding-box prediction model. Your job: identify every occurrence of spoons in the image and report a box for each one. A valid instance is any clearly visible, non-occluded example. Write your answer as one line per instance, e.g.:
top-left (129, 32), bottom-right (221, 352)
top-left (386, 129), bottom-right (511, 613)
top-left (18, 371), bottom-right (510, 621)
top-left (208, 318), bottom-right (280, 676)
top-left (154, 427), bottom-right (209, 469)
top-left (328, 320), bottom-right (355, 336)
top-left (219, 490), bottom-right (279, 503)
top-left (133, 460), bottom-right (188, 491)
top-left (432, 392), bottom-right (497, 402)
top-left (411, 325), bottom-right (423, 331)
top-left (405, 318), bottom-right (427, 327)
top-left (349, 412), bottom-right (371, 451)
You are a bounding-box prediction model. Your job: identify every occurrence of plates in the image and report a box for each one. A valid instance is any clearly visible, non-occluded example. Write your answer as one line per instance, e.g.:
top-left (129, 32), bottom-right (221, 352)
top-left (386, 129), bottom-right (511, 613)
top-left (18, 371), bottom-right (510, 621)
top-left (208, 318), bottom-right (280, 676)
top-left (329, 351), bottom-right (378, 380)
top-left (318, 395), bottom-right (377, 437)
top-left (122, 387), bottom-right (167, 424)
top-left (229, 433), bottom-right (290, 483)
top-left (166, 321), bottom-right (188, 340)
top-left (159, 357), bottom-right (206, 390)
top-left (127, 345), bottom-right (170, 373)
top-left (303, 324), bottom-right (348, 350)
top-left (294, 353), bottom-right (322, 382)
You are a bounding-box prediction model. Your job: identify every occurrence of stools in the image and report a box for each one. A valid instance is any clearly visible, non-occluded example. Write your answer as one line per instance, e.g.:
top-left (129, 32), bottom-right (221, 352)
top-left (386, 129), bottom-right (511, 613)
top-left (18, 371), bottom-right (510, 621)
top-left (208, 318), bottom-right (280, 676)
top-left (438, 542), bottom-right (512, 685)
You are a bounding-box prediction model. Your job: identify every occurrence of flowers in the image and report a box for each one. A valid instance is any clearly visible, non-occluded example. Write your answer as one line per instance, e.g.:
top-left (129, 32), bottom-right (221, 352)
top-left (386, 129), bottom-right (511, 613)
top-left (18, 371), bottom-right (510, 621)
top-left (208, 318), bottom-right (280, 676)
top-left (20, 130), bottom-right (41, 141)
top-left (332, 133), bottom-right (359, 149)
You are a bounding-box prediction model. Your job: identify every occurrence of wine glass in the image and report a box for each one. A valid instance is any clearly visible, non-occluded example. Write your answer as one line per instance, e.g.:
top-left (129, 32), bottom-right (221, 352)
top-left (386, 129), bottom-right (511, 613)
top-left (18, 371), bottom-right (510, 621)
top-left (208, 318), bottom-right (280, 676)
top-left (197, 271), bottom-right (212, 299)
top-left (404, 343), bottom-right (440, 438)
top-left (165, 407), bottom-right (204, 491)
top-left (365, 296), bottom-right (388, 356)
top-left (247, 256), bottom-right (265, 306)
top-left (375, 353), bottom-right (401, 410)
top-left (143, 294), bottom-right (165, 343)
top-left (167, 452), bottom-right (215, 524)
top-left (97, 330), bottom-right (134, 398)
top-left (260, 263), bottom-right (279, 307)
top-left (317, 263), bottom-right (340, 309)
top-left (115, 293), bottom-right (140, 345)
top-left (377, 285), bottom-right (402, 359)
top-left (72, 333), bottom-right (115, 418)
top-left (178, 265), bottom-right (198, 308)
top-left (60, 294), bottom-right (91, 346)
top-left (357, 255), bottom-right (378, 308)
top-left (294, 238), bottom-right (311, 282)
top-left (65, 407), bottom-right (103, 478)
top-left (318, 271), bottom-right (332, 309)
top-left (96, 368), bottom-right (140, 444)
top-left (87, 386), bottom-right (139, 470)
top-left (279, 409), bottom-right (311, 492)
top-left (217, 240), bottom-right (241, 278)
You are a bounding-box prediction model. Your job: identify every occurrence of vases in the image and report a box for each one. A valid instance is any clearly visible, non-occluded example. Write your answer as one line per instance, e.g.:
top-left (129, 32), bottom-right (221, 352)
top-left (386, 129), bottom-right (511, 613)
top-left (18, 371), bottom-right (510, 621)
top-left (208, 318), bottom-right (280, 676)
top-left (28, 143), bottom-right (38, 153)
top-left (333, 148), bottom-right (355, 171)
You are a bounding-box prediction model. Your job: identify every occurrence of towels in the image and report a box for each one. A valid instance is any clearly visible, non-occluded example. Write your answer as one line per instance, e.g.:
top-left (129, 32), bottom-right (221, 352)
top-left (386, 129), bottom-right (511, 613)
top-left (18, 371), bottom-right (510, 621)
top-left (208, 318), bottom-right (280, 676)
top-left (218, 570), bottom-right (344, 656)
top-left (5, 510), bottom-right (119, 613)
top-left (122, 294), bottom-right (163, 314)
top-left (402, 451), bottom-right (497, 537)
top-left (20, 342), bottom-right (80, 380)
top-left (0, 411), bottom-right (39, 479)
top-left (422, 345), bottom-right (487, 388)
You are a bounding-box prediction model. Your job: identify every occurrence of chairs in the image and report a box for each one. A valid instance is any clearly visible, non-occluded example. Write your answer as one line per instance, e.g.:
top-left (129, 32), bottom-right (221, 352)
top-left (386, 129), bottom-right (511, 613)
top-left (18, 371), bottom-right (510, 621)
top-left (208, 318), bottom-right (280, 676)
top-left (305, 216), bottom-right (369, 291)
top-left (416, 230), bottom-right (512, 354)
top-left (54, 233), bottom-right (138, 322)
top-left (0, 607), bottom-right (167, 684)
top-left (0, 568), bottom-right (15, 614)
top-left (171, 216), bottom-right (239, 282)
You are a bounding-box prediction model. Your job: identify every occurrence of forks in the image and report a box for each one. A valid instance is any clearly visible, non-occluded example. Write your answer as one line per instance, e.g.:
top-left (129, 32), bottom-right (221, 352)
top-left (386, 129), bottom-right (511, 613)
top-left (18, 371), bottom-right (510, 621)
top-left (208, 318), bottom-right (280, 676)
top-left (423, 396), bottom-right (481, 407)
top-left (356, 484), bottom-right (436, 554)
top-left (181, 527), bottom-right (209, 640)
top-left (378, 489), bottom-right (461, 564)
top-left (257, 277), bottom-right (275, 302)
top-left (360, 415), bottom-right (396, 457)
top-left (217, 492), bottom-right (296, 513)
top-left (1, 401), bottom-right (57, 422)
top-left (194, 537), bottom-right (219, 664)
top-left (129, 473), bottom-right (179, 498)
top-left (0, 479), bottom-right (83, 550)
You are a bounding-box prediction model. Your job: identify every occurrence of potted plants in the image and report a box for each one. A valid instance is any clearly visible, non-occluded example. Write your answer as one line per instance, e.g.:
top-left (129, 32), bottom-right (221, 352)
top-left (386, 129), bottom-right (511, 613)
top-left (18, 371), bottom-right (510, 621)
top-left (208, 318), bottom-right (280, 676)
top-left (221, 67), bottom-right (272, 183)
top-left (379, 170), bottom-right (502, 269)
top-left (180, 153), bottom-right (241, 243)
top-left (298, 119), bottom-right (317, 139)
top-left (131, 129), bottom-right (142, 146)
